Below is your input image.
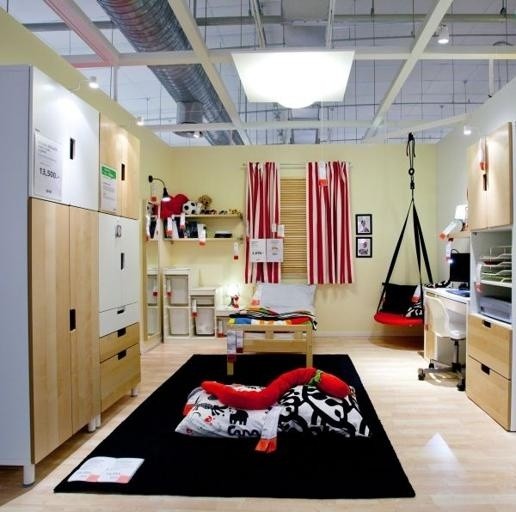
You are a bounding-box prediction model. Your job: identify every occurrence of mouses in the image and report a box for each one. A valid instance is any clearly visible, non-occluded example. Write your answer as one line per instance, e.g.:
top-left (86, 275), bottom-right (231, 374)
top-left (464, 292), bottom-right (469, 297)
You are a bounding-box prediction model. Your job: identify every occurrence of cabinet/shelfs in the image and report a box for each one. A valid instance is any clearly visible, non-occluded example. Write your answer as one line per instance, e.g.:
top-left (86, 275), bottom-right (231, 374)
top-left (98, 113), bottom-right (140, 223)
top-left (465, 121), bottom-right (516, 432)
top-left (0, 65), bottom-right (99, 211)
top-left (98, 213), bottom-right (141, 415)
top-left (1, 197), bottom-right (100, 464)
top-left (147, 210), bottom-right (242, 242)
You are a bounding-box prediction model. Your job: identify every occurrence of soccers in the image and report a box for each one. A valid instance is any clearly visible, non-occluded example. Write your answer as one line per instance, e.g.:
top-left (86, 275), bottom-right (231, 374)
top-left (183, 201), bottom-right (197, 215)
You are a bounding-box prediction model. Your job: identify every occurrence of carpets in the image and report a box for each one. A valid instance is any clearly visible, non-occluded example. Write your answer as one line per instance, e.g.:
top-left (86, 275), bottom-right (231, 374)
top-left (47, 351), bottom-right (422, 500)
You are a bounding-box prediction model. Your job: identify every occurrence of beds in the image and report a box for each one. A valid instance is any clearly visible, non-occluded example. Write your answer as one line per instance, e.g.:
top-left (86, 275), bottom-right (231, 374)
top-left (226, 282), bottom-right (319, 376)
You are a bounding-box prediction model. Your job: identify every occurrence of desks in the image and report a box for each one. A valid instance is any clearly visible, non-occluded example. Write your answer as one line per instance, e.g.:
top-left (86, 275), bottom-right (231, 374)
top-left (421, 282), bottom-right (466, 366)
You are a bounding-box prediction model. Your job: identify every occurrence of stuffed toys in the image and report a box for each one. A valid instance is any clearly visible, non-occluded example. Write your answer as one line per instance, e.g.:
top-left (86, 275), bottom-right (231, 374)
top-left (199, 194), bottom-right (213, 215)
top-left (201, 367), bottom-right (352, 410)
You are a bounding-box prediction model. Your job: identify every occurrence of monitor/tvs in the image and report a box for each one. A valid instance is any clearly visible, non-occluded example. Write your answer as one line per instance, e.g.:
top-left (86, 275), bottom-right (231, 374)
top-left (449, 252), bottom-right (470, 290)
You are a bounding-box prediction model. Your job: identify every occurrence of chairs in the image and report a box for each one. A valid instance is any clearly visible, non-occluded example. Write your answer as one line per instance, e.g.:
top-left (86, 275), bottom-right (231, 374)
top-left (418, 294), bottom-right (469, 389)
top-left (373, 311), bottom-right (423, 328)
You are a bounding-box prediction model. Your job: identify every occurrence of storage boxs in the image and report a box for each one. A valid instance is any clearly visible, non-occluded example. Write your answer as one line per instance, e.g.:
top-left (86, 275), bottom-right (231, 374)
top-left (165, 271), bottom-right (219, 339)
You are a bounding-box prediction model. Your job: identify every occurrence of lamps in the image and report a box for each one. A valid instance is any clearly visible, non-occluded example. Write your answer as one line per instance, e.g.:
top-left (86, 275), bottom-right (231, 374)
top-left (437, 27), bottom-right (453, 45)
top-left (460, 122), bottom-right (472, 135)
top-left (232, 50), bottom-right (357, 110)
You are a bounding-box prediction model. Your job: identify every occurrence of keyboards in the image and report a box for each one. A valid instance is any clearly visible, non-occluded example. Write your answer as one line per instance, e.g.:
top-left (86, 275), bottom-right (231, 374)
top-left (446, 289), bottom-right (465, 296)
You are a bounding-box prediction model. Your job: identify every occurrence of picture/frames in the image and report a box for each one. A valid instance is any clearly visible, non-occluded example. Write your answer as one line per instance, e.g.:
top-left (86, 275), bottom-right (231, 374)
top-left (355, 213), bottom-right (373, 259)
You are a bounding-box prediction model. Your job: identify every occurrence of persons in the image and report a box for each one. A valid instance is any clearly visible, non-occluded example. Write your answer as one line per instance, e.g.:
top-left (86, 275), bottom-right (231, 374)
top-left (358, 241), bottom-right (369, 255)
top-left (359, 218), bottom-right (369, 232)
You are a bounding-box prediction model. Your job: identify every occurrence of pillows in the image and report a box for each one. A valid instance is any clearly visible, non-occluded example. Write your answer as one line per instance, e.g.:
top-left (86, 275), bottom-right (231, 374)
top-left (379, 282), bottom-right (417, 313)
top-left (175, 367), bottom-right (372, 452)
